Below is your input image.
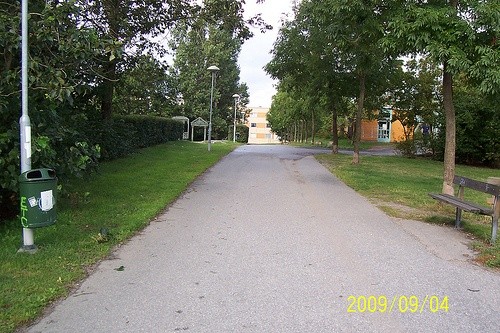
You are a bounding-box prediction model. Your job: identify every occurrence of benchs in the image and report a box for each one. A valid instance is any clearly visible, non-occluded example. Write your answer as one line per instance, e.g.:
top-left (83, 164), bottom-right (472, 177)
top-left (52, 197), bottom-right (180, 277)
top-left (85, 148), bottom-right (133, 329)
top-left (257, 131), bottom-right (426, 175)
top-left (428, 174), bottom-right (500, 245)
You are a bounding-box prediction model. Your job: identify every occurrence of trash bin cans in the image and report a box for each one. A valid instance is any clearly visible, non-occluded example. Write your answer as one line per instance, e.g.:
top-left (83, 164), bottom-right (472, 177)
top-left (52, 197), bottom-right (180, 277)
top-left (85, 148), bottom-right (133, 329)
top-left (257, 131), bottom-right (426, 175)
top-left (17, 168), bottom-right (57, 229)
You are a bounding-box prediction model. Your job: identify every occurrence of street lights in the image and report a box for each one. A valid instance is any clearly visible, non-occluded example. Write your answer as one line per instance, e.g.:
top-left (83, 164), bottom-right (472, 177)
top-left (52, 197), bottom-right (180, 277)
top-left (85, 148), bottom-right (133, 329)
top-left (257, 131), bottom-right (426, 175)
top-left (232, 94), bottom-right (240, 142)
top-left (206, 65), bottom-right (221, 153)
